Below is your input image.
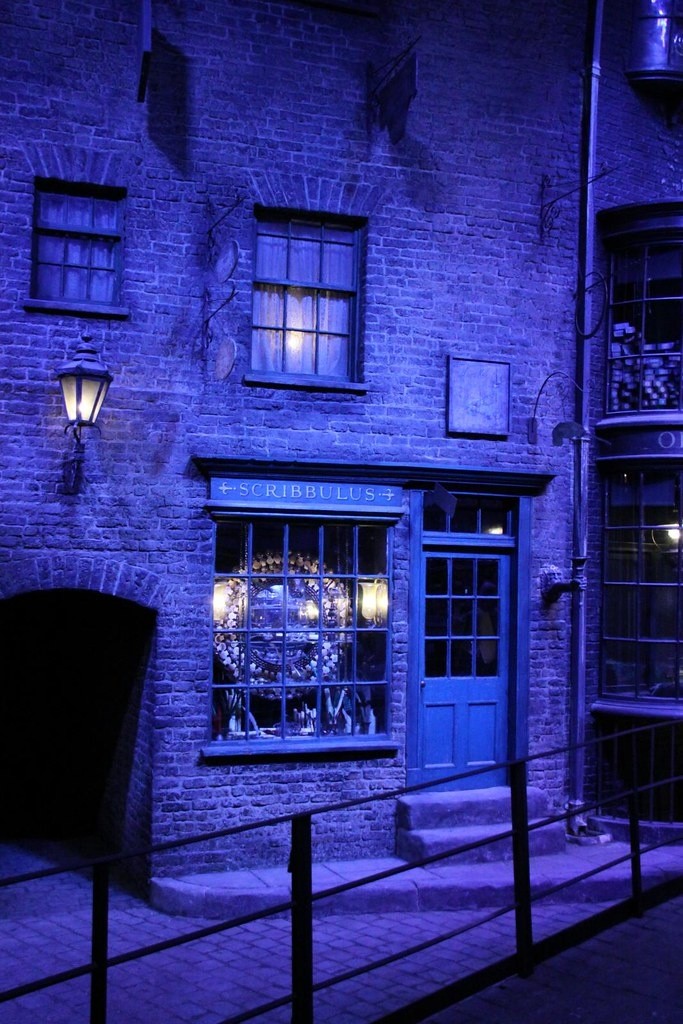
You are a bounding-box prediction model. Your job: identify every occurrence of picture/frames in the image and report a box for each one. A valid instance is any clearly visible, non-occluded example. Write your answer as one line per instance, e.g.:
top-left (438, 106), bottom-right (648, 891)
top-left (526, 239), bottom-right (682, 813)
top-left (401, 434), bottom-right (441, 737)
top-left (446, 355), bottom-right (513, 436)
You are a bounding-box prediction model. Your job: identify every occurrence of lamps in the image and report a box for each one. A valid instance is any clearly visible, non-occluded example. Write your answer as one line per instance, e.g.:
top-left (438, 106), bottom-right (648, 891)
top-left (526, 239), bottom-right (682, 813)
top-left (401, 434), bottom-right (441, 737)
top-left (359, 573), bottom-right (388, 629)
top-left (528, 371), bottom-right (613, 447)
top-left (53, 323), bottom-right (115, 495)
top-left (213, 583), bottom-right (245, 622)
